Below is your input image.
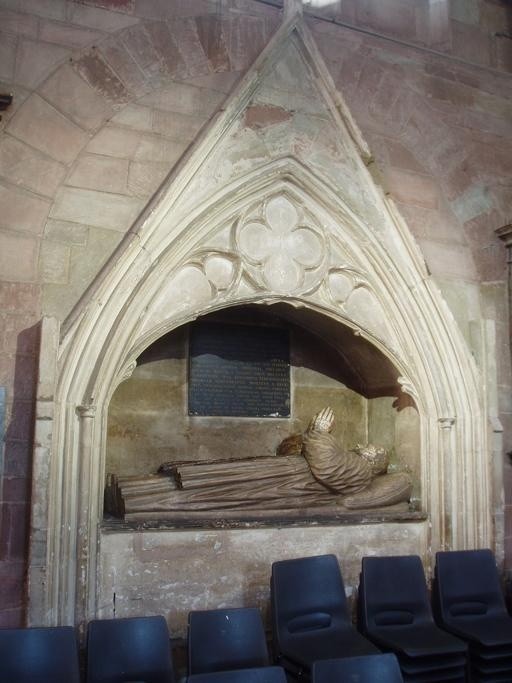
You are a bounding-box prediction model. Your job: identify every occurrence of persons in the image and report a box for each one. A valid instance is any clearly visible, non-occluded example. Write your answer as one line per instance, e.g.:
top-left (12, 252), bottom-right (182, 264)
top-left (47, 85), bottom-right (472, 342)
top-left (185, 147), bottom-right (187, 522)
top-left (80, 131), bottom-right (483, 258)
top-left (104, 404), bottom-right (393, 524)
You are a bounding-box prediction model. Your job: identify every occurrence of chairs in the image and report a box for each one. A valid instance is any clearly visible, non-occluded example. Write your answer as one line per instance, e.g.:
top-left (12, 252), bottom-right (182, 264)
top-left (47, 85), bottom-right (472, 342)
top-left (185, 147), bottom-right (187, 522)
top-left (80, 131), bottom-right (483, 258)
top-left (0, 549), bottom-right (512, 683)
